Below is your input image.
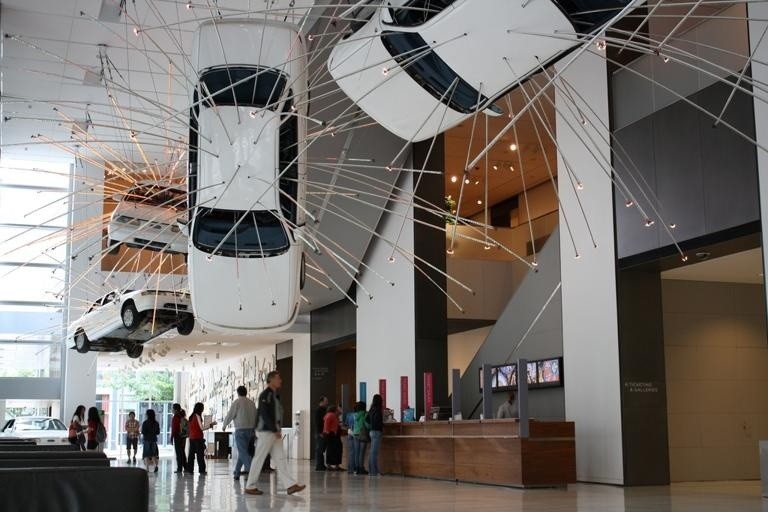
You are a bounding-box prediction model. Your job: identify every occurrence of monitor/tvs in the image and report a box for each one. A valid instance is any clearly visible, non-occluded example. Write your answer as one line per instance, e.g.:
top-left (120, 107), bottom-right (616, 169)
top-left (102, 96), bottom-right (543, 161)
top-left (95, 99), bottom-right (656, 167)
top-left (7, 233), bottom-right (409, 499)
top-left (537, 355), bottom-right (562, 389)
top-left (497, 363), bottom-right (518, 391)
top-left (479, 366), bottom-right (498, 392)
top-left (516, 360), bottom-right (537, 389)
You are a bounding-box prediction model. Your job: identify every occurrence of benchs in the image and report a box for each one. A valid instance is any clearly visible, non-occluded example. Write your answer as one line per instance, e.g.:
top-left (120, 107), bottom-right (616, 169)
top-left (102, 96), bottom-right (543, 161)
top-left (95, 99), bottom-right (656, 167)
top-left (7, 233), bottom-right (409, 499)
top-left (0, 436), bottom-right (149, 512)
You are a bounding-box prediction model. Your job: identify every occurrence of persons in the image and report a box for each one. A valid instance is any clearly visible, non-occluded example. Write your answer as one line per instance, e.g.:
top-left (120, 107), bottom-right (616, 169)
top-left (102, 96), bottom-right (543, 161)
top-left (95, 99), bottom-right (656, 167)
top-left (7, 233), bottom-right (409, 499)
top-left (68, 405), bottom-right (88, 451)
top-left (141, 409), bottom-right (160, 473)
top-left (125, 412), bottom-right (140, 463)
top-left (315, 394), bottom-right (385, 477)
top-left (245, 371), bottom-right (306, 495)
top-left (497, 391), bottom-right (519, 418)
top-left (148, 443), bottom-right (159, 464)
top-left (222, 386), bottom-right (258, 480)
top-left (188, 402), bottom-right (217, 475)
top-left (84, 407), bottom-right (100, 451)
top-left (171, 403), bottom-right (188, 473)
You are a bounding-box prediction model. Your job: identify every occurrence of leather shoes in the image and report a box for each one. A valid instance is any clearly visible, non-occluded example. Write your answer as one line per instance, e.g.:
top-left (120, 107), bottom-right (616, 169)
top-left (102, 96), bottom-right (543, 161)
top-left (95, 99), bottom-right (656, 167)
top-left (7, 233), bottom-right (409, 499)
top-left (327, 464), bottom-right (369, 475)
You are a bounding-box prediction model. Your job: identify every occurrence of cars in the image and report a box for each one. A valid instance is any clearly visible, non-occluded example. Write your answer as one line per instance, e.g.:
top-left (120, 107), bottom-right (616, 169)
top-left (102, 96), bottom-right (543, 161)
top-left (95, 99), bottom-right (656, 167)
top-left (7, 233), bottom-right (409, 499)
top-left (104, 180), bottom-right (189, 262)
top-left (1, 416), bottom-right (70, 445)
top-left (67, 287), bottom-right (195, 359)
top-left (188, 19), bottom-right (311, 337)
top-left (326, 0), bottom-right (648, 145)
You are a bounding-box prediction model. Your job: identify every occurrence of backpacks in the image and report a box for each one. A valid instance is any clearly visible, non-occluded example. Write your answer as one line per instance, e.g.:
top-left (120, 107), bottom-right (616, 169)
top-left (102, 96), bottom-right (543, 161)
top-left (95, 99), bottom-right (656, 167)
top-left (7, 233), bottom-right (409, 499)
top-left (179, 416), bottom-right (189, 439)
top-left (95, 422), bottom-right (107, 444)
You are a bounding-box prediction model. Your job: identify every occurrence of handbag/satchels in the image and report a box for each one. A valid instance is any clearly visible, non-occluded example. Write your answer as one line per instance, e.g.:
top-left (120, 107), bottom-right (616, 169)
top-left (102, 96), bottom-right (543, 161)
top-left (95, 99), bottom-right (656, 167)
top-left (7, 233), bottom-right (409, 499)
top-left (356, 424), bottom-right (370, 443)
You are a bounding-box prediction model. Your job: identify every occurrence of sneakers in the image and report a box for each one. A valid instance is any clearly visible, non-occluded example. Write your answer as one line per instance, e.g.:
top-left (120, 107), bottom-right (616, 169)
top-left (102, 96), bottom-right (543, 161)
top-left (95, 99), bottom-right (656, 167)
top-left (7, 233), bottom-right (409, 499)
top-left (243, 487), bottom-right (265, 496)
top-left (286, 483), bottom-right (307, 497)
